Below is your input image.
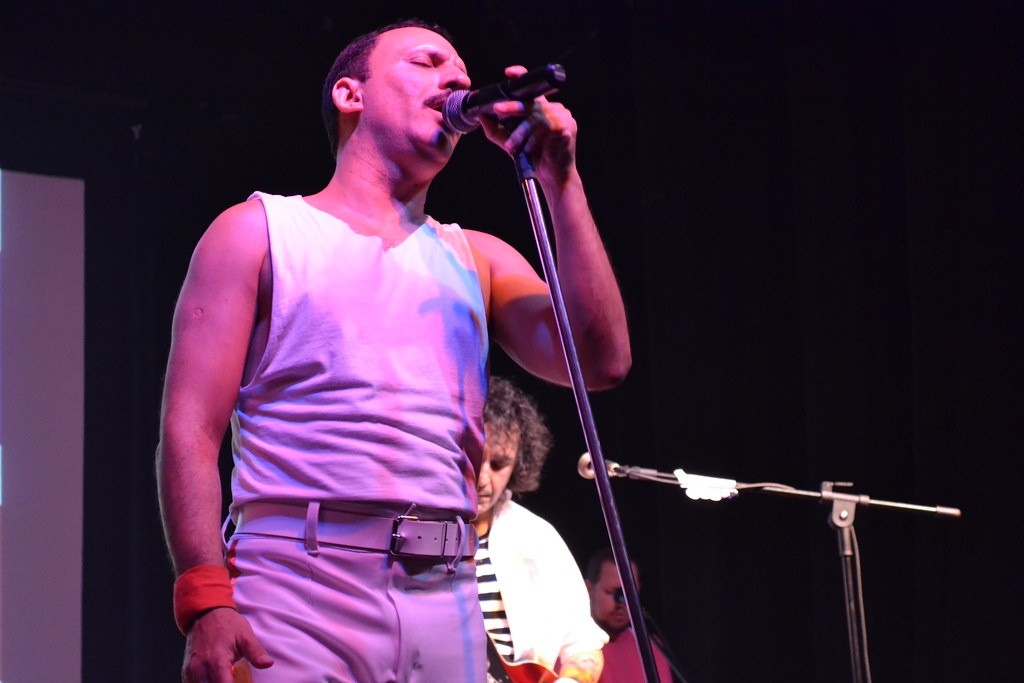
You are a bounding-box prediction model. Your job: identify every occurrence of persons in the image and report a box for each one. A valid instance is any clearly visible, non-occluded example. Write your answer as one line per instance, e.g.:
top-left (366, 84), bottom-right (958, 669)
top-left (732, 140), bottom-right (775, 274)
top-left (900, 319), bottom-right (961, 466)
top-left (583, 552), bottom-right (672, 683)
top-left (157, 25), bottom-right (635, 683)
top-left (475, 374), bottom-right (610, 683)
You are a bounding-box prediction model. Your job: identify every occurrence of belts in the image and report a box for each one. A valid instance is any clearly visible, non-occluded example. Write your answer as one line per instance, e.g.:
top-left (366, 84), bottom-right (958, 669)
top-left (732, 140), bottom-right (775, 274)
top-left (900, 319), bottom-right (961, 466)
top-left (233, 501), bottom-right (479, 559)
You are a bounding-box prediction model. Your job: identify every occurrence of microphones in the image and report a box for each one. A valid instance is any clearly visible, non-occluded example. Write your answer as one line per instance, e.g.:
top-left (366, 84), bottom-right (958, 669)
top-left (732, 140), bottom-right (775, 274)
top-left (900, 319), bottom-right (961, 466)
top-left (577, 452), bottom-right (628, 480)
top-left (441, 62), bottom-right (569, 136)
